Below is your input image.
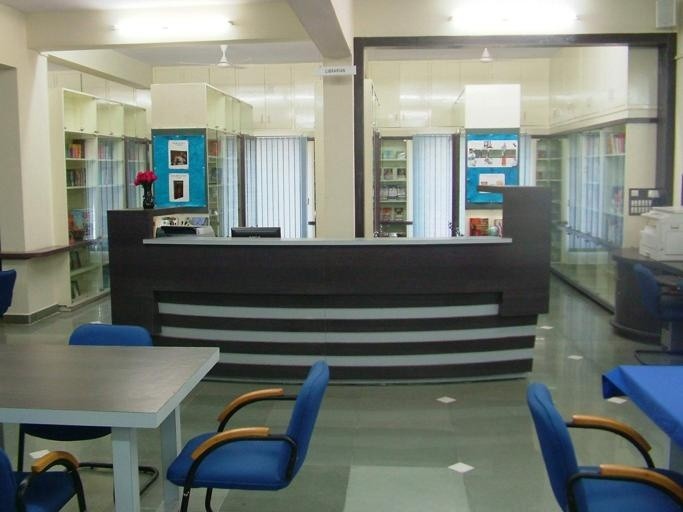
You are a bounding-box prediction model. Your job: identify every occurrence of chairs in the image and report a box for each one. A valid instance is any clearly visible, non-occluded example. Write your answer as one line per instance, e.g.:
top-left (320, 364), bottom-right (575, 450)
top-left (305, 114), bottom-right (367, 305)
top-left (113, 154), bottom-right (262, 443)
top-left (18, 324), bottom-right (159, 506)
top-left (525, 381), bottom-right (683, 512)
top-left (0, 450), bottom-right (86, 512)
top-left (0, 269), bottom-right (16, 319)
top-left (164, 361), bottom-right (330, 512)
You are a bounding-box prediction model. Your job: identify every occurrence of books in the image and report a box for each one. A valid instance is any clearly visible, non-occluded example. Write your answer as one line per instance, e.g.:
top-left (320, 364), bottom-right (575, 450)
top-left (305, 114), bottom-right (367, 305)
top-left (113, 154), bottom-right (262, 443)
top-left (602, 134), bottom-right (625, 153)
top-left (381, 148), bottom-right (405, 237)
top-left (65, 134), bottom-right (88, 300)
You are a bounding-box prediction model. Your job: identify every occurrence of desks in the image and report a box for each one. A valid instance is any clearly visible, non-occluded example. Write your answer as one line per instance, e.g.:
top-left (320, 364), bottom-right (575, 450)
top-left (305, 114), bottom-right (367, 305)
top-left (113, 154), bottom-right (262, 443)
top-left (109, 185), bottom-right (550, 383)
top-left (1, 339), bottom-right (219, 512)
top-left (620, 364), bottom-right (683, 481)
top-left (609, 247), bottom-right (682, 350)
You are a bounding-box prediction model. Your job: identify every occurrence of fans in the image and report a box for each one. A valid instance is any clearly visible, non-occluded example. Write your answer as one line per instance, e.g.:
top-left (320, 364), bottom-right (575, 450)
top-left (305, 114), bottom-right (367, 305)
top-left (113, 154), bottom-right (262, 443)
top-left (445, 47), bottom-right (531, 64)
top-left (179, 45), bottom-right (267, 71)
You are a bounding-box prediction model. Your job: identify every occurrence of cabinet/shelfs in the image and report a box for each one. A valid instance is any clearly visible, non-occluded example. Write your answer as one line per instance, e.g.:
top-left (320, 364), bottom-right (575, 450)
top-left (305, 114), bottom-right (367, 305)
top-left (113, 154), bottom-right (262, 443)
top-left (374, 129), bottom-right (457, 239)
top-left (151, 85), bottom-right (256, 238)
top-left (521, 116), bottom-right (659, 318)
top-left (61, 89), bottom-right (151, 311)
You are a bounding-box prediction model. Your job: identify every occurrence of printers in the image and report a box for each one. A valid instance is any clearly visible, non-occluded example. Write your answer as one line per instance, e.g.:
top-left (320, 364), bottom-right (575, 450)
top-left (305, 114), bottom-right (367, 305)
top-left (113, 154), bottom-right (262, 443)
top-left (639, 206), bottom-right (683, 262)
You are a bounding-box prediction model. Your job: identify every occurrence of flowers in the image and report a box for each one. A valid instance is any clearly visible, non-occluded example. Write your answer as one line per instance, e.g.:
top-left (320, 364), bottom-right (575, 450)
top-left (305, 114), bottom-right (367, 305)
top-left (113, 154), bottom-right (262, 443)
top-left (134, 170), bottom-right (158, 186)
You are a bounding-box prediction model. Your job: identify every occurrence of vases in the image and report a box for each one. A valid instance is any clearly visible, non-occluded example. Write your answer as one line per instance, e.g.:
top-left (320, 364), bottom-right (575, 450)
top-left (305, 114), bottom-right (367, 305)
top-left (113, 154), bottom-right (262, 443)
top-left (143, 186), bottom-right (154, 210)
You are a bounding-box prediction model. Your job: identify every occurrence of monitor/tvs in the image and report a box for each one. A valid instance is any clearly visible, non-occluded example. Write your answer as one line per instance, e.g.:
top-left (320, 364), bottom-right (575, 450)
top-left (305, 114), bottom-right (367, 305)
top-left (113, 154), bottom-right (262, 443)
top-left (231, 226), bottom-right (281, 237)
